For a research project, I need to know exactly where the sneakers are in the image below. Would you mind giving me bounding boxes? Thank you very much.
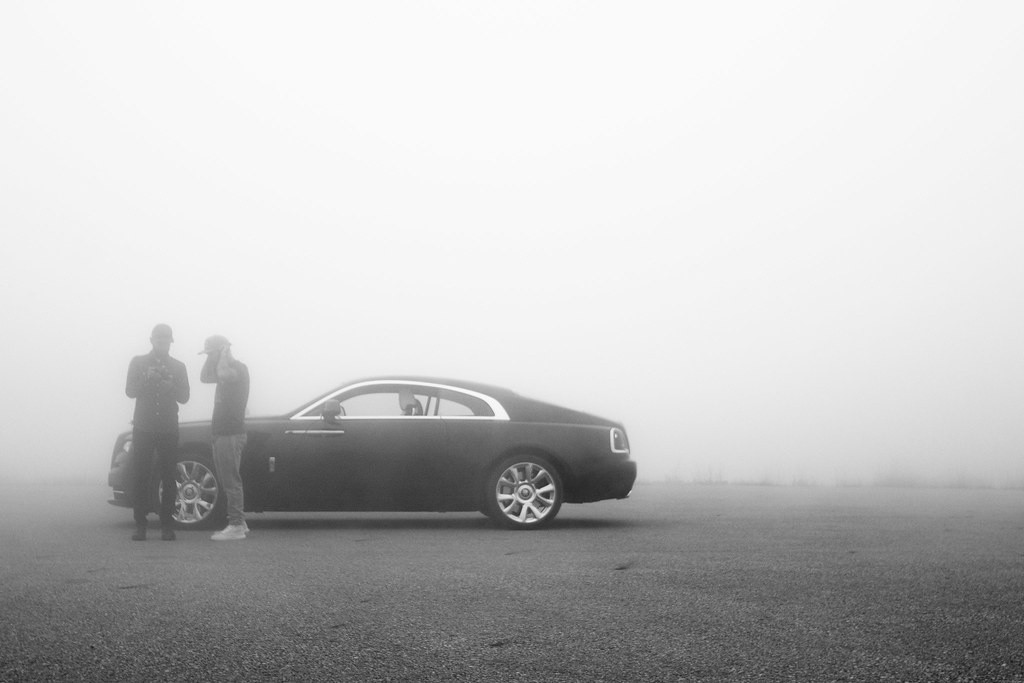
[210,521,249,540]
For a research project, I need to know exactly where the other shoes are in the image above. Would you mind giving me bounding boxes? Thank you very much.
[131,532,145,540]
[162,530,176,540]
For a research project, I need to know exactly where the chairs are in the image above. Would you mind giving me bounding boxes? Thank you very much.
[398,388,417,416]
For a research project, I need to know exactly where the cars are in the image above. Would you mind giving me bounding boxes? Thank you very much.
[111,375,639,533]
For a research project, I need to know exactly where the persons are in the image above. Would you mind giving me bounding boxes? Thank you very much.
[125,324,191,539]
[197,336,249,538]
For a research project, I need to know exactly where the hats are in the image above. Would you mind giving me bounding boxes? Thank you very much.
[151,324,173,342]
[198,334,231,355]
